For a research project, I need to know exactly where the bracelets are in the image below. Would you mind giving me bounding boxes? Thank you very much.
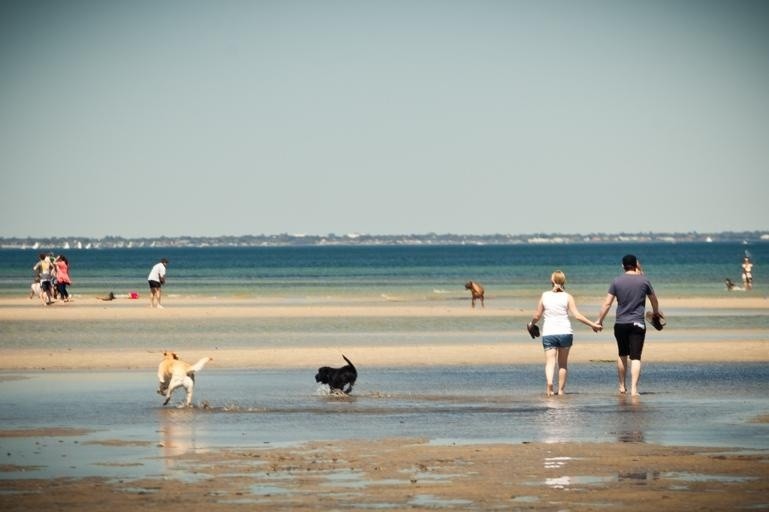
[531,320,536,326]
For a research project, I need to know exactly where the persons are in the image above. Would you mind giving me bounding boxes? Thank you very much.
[741,258,755,291]
[147,258,169,309]
[725,278,747,291]
[95,291,140,301]
[527,269,604,396]
[590,254,665,397]
[28,250,74,307]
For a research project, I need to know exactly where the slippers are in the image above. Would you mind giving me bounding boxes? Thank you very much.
[646,309,666,330]
[527,321,540,338]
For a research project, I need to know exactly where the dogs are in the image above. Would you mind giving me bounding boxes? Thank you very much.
[463,279,487,309]
[314,353,359,398]
[153,348,211,407]
[30,280,57,304]
